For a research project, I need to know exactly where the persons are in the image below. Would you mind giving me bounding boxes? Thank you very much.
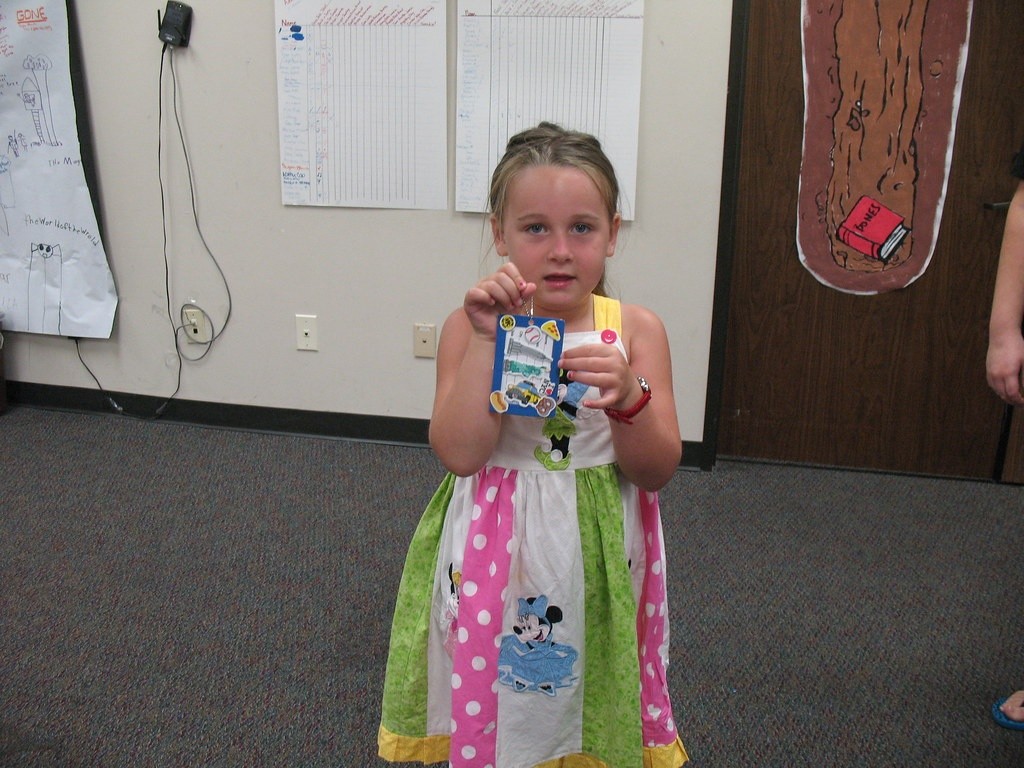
[983,149,1024,732]
[373,121,694,767]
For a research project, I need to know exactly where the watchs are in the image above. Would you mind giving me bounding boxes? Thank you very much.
[602,375,653,426]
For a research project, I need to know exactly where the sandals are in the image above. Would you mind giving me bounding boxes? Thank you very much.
[992,690,1024,730]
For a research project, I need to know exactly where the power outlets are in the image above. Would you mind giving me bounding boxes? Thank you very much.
[182,310,207,344]
[296,315,318,350]
[414,323,436,358]
